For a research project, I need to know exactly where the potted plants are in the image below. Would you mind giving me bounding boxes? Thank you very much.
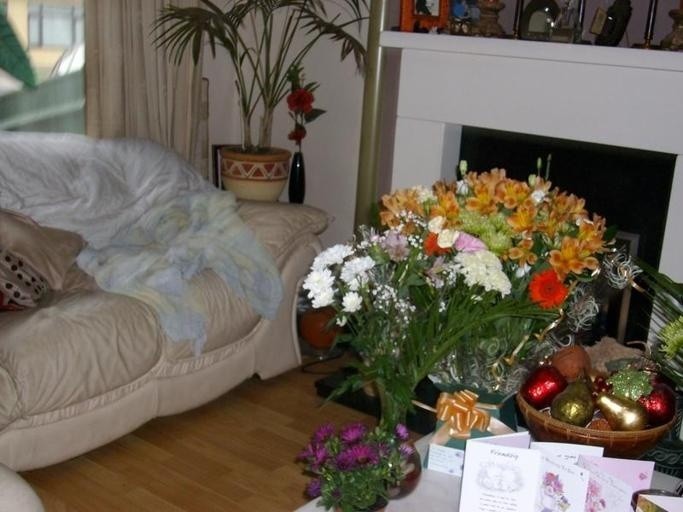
[152,1,374,204]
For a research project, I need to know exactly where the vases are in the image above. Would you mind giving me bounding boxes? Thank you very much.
[289,150,306,204]
[374,424,424,501]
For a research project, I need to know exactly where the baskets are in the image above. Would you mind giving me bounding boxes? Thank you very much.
[517,383,679,458]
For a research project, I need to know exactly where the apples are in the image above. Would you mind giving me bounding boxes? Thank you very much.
[640,386,675,424]
[521,366,567,410]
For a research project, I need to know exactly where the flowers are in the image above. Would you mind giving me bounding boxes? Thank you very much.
[306,211,542,430]
[279,64,317,149]
[295,419,415,510]
[629,255,682,388]
[381,156,614,467]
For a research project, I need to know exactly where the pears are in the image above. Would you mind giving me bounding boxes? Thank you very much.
[593,389,649,430]
[551,379,594,426]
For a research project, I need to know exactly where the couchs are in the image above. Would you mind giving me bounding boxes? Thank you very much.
[1,126,326,476]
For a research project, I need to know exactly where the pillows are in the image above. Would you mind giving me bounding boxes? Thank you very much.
[1,203,94,304]
